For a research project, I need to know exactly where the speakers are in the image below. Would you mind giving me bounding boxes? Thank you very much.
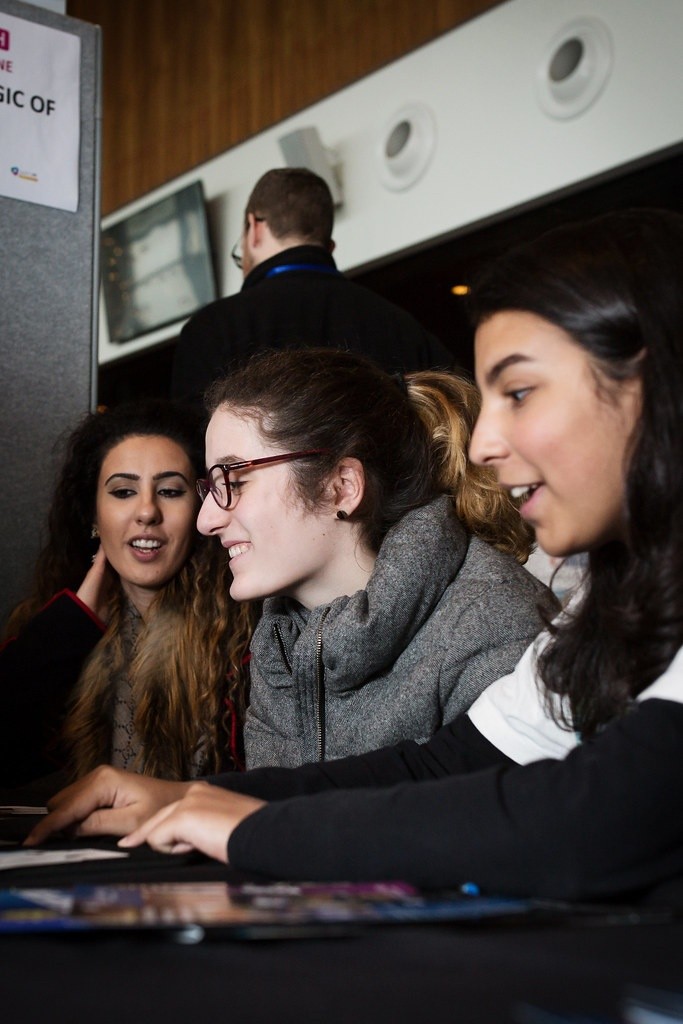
[279,128,344,206]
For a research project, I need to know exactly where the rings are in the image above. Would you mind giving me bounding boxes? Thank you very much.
[90,554,98,564]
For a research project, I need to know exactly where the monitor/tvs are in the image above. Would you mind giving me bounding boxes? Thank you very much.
[100,180,221,344]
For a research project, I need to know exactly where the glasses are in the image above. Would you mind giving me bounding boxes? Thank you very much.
[195,448,333,510]
[231,216,266,269]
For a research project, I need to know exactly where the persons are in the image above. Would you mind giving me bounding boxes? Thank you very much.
[1,206,682,916]
[156,168,453,439]
